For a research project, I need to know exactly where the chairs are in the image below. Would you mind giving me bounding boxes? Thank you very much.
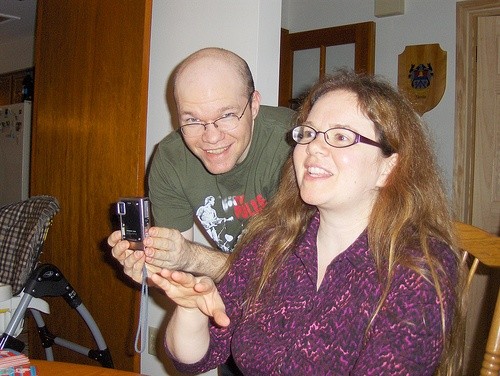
[449,222,500,376]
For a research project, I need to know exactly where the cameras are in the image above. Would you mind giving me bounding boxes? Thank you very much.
[118,197,151,242]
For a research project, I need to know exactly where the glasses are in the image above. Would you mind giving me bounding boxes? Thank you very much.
[178,93,253,137]
[291,123,386,150]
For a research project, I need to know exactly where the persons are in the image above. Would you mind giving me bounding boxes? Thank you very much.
[152,69,468,376]
[107,47,298,376]
